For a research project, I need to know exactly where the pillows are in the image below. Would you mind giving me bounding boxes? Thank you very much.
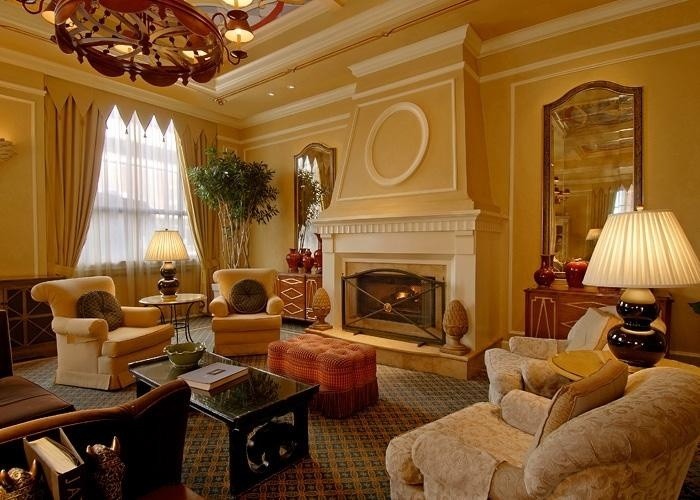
[227,279,268,313]
[534,359,627,446]
[563,307,623,351]
[76,291,124,332]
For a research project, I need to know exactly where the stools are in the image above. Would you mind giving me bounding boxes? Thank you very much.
[267,333,379,418]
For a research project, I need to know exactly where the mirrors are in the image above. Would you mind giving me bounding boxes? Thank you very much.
[542,79,643,278]
[294,143,338,258]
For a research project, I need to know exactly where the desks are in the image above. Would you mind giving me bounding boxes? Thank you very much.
[137,486,204,500]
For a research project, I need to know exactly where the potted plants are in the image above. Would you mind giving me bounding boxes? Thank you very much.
[294,163,327,249]
[188,146,279,299]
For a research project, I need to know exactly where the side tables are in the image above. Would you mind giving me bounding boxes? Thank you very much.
[139,294,207,344]
[550,351,697,382]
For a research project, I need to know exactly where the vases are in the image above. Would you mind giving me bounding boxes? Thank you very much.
[285,247,322,275]
[564,262,587,287]
[533,255,555,289]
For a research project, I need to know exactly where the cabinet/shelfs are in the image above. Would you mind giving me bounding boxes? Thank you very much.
[524,287,675,359]
[277,273,322,323]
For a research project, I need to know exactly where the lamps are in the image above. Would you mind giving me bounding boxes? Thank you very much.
[554,176,570,204]
[581,208,700,367]
[587,229,602,246]
[144,228,190,296]
[15,1,252,86]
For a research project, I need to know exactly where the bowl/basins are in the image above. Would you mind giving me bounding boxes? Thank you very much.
[163,342,206,366]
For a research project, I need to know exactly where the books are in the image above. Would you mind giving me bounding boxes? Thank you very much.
[21,427,87,500]
[176,361,249,390]
[189,375,251,397]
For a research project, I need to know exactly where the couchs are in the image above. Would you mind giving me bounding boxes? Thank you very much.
[31,276,175,390]
[210,269,284,356]
[383,361,700,499]
[483,304,668,402]
[1,310,190,487]
